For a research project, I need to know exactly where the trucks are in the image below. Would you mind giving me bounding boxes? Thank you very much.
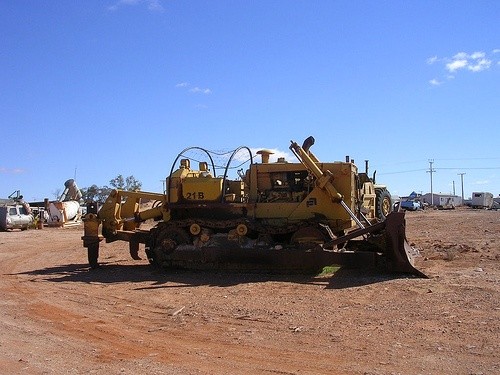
[401,196,422,211]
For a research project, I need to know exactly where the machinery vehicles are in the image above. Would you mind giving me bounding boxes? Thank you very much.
[81,136,429,279]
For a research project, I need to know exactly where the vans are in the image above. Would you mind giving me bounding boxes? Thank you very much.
[0,204,35,232]
